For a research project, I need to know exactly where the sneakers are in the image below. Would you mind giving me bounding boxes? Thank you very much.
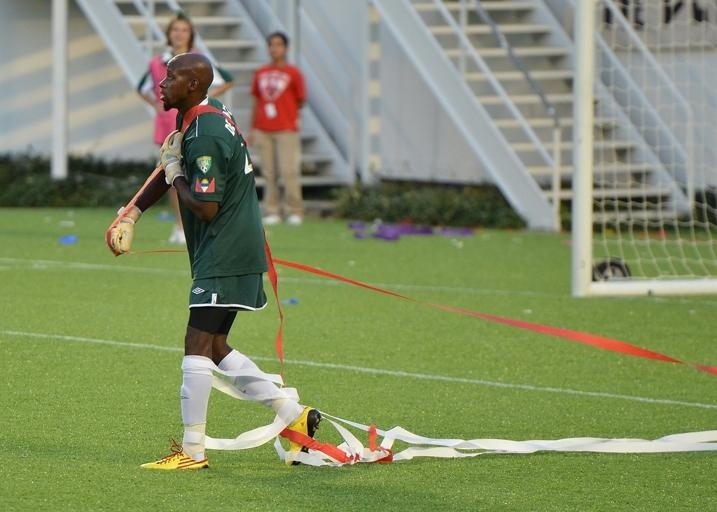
[286,405,321,466]
[262,213,303,225]
[170,226,185,243]
[141,450,209,470]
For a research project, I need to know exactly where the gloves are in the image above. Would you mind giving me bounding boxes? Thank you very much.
[106,204,143,257]
[159,129,184,187]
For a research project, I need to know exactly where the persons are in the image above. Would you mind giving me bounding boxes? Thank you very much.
[134,15,235,242]
[105,53,322,470]
[246,32,308,224]
